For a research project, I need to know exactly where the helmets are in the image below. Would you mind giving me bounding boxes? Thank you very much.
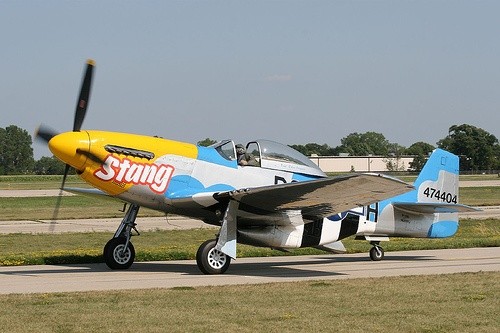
[233,144,246,155]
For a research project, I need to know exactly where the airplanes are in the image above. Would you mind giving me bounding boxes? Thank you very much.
[35,59,482,275]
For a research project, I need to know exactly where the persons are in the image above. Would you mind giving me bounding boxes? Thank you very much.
[235,144,260,167]
